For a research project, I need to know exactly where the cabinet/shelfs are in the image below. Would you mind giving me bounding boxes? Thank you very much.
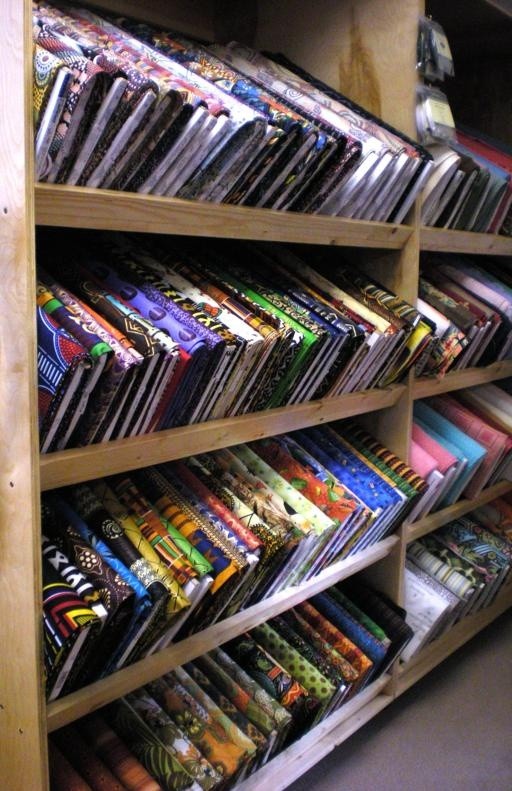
[0,0,511,791]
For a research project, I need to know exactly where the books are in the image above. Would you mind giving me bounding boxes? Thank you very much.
[44,378,511,791]
[36,226,510,458]
[30,1,512,240]
